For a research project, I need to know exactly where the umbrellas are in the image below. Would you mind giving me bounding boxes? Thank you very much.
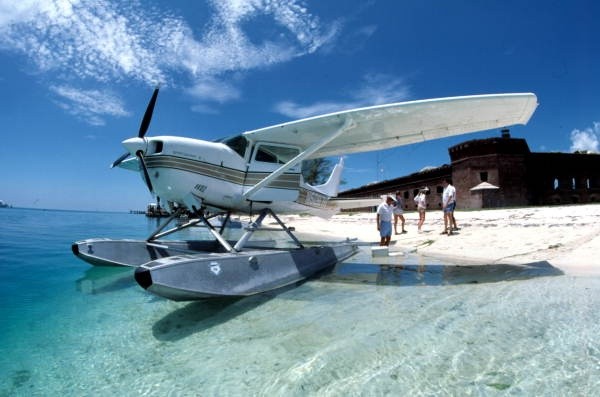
[469,182,500,208]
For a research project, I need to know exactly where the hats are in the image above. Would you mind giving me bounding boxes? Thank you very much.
[388,195,398,202]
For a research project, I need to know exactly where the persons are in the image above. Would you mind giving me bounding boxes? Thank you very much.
[393,190,408,235]
[377,194,396,247]
[447,179,459,231]
[413,185,430,233]
[440,178,454,236]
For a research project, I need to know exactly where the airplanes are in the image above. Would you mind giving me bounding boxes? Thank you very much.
[72,81,538,303]
